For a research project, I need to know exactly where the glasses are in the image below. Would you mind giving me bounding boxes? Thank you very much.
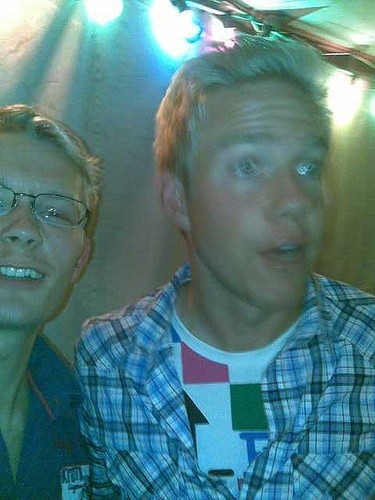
[1,185,91,230]
